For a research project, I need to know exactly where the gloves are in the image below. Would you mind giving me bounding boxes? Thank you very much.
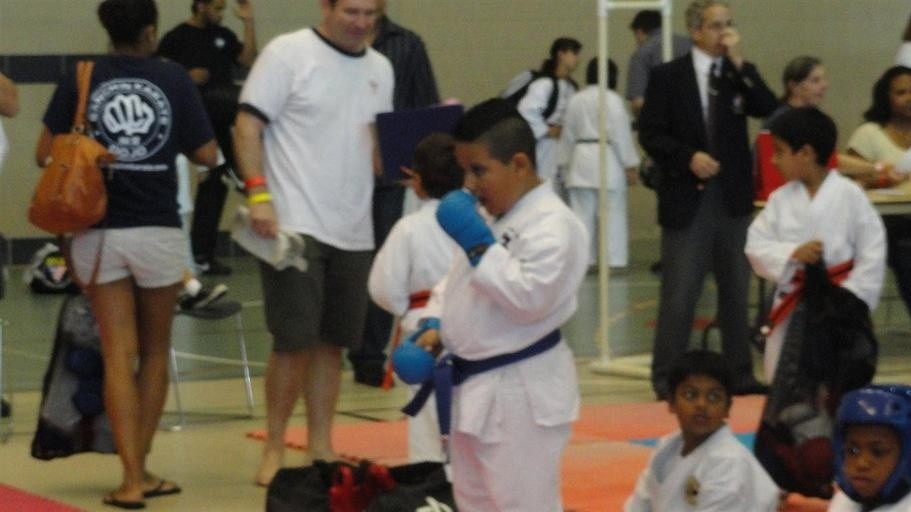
[432,187,498,272]
[390,314,444,389]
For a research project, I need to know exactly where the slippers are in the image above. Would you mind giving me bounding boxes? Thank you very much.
[103,489,146,509]
[144,470,183,497]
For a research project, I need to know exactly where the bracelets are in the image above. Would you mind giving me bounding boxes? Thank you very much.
[244,191,275,208]
[874,159,884,173]
[242,176,271,188]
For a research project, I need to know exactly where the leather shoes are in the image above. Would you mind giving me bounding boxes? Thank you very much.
[732,382,772,396]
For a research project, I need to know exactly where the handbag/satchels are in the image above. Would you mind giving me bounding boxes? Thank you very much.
[22,59,118,235]
[25,284,128,461]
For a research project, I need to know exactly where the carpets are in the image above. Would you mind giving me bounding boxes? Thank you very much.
[0,483,86,512]
[245,394,840,512]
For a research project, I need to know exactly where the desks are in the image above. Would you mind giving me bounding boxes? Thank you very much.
[754,190,911,318]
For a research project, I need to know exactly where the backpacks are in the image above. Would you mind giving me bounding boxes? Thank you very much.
[498,70,561,120]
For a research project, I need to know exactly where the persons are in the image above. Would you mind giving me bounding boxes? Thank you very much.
[2,0,911,512]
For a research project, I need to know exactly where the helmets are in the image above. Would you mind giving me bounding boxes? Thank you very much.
[831,382,910,511]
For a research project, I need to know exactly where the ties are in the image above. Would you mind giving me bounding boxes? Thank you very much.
[705,59,724,148]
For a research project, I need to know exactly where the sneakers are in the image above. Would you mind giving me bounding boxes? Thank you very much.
[342,345,397,390]
[180,281,233,314]
[253,441,290,490]
[302,441,348,465]
[191,252,238,282]
